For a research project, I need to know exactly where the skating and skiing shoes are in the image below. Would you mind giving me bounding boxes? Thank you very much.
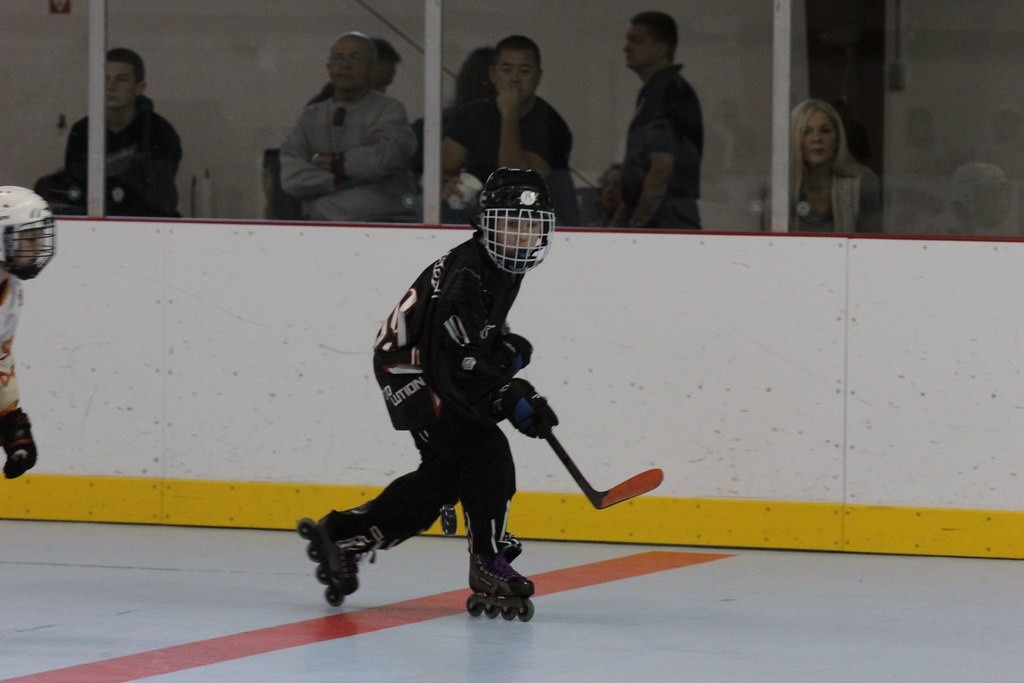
[466,552,535,622]
[298,509,359,606]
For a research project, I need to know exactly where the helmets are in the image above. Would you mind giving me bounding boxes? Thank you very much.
[478,168,555,274]
[0,185,56,280]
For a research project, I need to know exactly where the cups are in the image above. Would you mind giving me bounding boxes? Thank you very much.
[448,172,483,211]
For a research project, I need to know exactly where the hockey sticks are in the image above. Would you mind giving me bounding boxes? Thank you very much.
[548,432,664,511]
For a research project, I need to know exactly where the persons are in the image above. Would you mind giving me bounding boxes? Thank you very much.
[263,41,401,218]
[440,35,580,224]
[606,11,704,230]
[63,47,181,217]
[789,99,882,233]
[414,47,494,173]
[295,166,560,621]
[601,164,623,213]
[279,30,419,222]
[1,185,55,478]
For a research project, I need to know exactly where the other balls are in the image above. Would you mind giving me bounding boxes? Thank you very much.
[440,503,458,537]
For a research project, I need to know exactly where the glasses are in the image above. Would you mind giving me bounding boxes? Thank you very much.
[329,55,371,65]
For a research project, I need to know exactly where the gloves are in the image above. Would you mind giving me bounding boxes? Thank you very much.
[481,334,533,377]
[0,408,38,479]
[496,378,558,439]
